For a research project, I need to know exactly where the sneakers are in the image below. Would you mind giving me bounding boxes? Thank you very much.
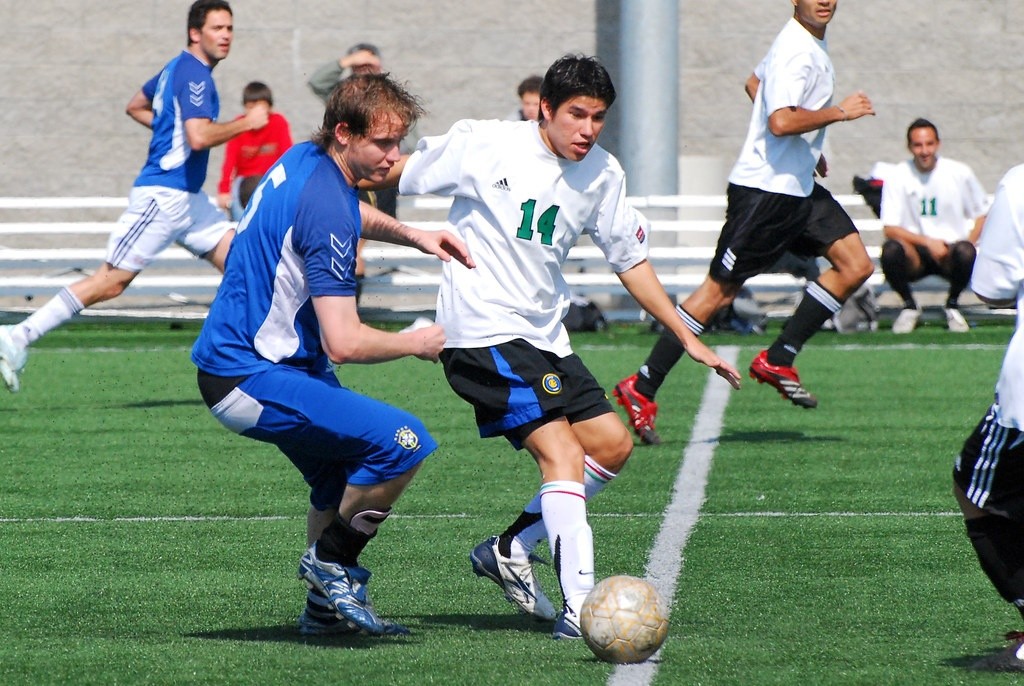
[551,610,584,640]
[893,304,922,336]
[613,372,662,447]
[943,305,968,334]
[297,612,399,637]
[0,325,27,392]
[296,539,385,635]
[748,351,817,408]
[973,630,1024,669]
[469,533,558,621]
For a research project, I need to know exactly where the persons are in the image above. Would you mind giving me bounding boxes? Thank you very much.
[0,0,268,395]
[952,163,1024,660]
[355,53,741,637]
[506,74,544,123]
[613,0,876,447]
[309,42,421,310]
[218,82,291,222]
[880,119,986,333]
[193,69,474,636]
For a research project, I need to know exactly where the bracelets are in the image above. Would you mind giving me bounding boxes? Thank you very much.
[834,104,848,121]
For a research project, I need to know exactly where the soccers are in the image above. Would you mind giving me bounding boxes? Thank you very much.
[579,575,669,664]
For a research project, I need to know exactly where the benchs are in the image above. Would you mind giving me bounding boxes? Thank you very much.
[0,195,994,291]
